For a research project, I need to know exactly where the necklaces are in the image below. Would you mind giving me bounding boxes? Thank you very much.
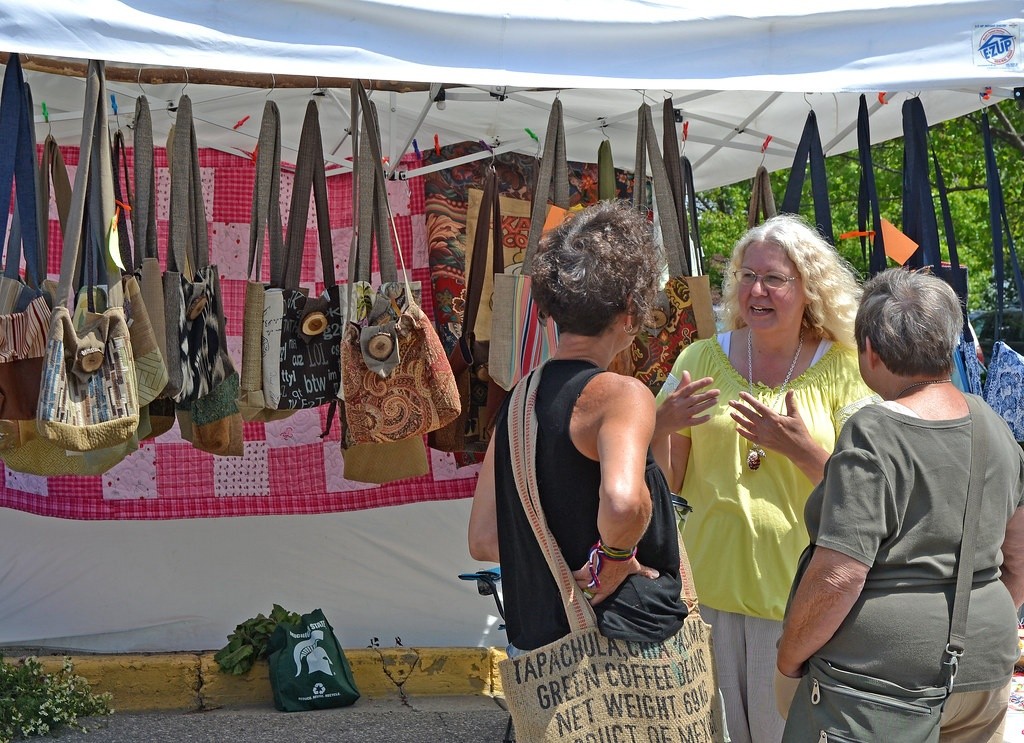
[891,379,953,401]
[748,327,804,469]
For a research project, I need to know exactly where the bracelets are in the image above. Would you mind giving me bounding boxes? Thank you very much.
[588,539,637,587]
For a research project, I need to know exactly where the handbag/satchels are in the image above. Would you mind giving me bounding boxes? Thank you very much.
[0,53,717,484]
[498,614,726,743]
[781,656,950,743]
[267,608,360,712]
[902,97,1024,442]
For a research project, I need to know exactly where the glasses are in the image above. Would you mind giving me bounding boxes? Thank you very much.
[732,267,801,289]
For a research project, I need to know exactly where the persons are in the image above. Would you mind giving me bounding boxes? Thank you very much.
[650,215,883,743]
[776,270,1024,743]
[468,201,689,655]
[941,263,985,361]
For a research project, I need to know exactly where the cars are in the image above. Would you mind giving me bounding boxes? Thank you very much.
[963,306,1024,357]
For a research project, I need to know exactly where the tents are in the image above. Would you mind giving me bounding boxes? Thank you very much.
[0,0,1024,654]
